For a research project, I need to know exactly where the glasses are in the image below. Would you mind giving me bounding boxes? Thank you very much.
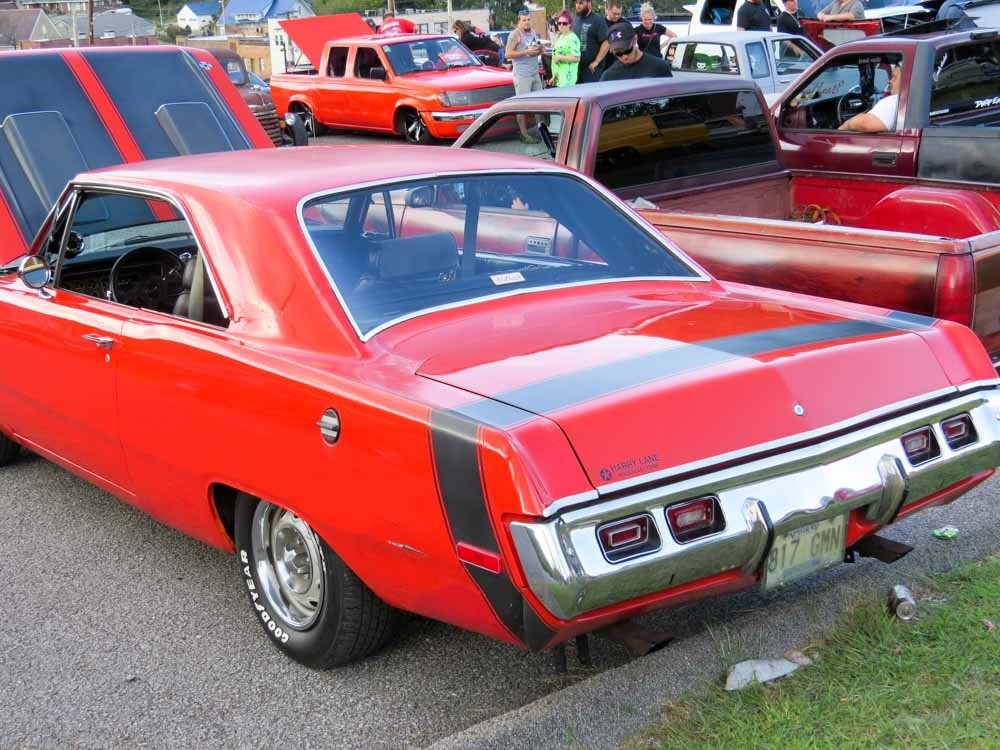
[454,31,456,33]
[614,42,635,57]
[558,21,568,26]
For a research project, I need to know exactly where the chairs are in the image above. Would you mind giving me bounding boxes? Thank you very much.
[409,56,437,71]
[170,252,224,320]
[365,230,460,277]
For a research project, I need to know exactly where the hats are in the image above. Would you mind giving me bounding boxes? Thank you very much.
[607,22,634,54]
[517,7,530,17]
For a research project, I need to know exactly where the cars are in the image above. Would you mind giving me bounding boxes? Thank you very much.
[267,12,539,144]
[438,0,1000,149]
[1,43,1000,672]
[202,45,311,147]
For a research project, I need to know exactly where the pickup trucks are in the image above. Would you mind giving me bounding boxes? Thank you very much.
[311,72,999,381]
[767,27,1000,188]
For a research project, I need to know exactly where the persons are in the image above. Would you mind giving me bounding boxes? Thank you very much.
[547,8,581,88]
[504,7,550,145]
[452,21,505,66]
[572,0,609,84]
[836,60,903,133]
[603,0,631,69]
[599,22,673,82]
[634,2,677,60]
[738,0,776,73]
[777,0,803,58]
[377,11,417,33]
[817,0,864,22]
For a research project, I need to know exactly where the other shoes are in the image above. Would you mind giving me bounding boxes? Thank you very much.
[520,135,538,144]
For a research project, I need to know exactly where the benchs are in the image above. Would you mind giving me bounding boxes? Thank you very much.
[354,262,607,288]
